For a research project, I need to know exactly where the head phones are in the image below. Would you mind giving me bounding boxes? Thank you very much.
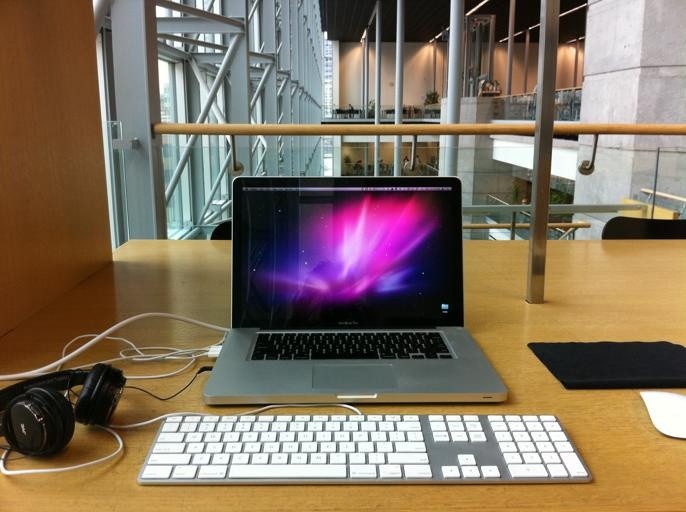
[0,364,127,457]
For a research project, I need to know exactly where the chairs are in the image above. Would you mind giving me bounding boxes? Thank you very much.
[329,102,422,119]
[602,214,685,239]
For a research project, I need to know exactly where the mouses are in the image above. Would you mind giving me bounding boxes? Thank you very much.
[639,389,685,437]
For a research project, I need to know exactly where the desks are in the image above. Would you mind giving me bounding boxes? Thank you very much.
[0,239,686,512]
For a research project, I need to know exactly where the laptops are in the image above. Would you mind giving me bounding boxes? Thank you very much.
[203,174,508,406]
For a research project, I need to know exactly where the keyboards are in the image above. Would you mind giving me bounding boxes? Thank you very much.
[138,412,592,484]
